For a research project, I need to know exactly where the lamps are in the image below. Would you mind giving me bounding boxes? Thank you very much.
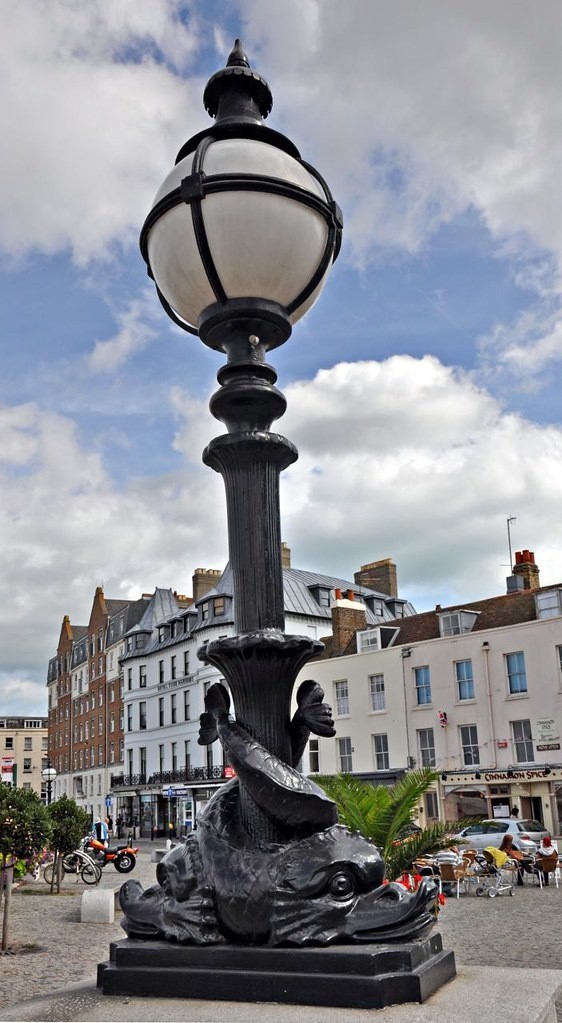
[441,768,449,780]
[544,762,553,774]
[475,768,484,779]
[507,764,514,777]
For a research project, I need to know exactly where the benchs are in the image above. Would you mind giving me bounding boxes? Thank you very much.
[151,849,169,861]
[79,881,124,925]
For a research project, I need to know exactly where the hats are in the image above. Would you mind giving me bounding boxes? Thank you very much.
[543,837,551,847]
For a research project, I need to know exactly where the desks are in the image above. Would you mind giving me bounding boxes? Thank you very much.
[519,857,534,864]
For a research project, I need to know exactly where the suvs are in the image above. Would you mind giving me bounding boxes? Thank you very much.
[453,818,551,854]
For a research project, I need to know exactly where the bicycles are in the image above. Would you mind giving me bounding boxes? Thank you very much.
[44,846,102,886]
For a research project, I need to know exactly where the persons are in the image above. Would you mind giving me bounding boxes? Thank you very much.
[93,817,108,845]
[499,835,558,886]
[413,846,462,896]
[105,815,113,847]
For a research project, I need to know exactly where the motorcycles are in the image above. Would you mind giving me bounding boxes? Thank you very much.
[62,835,140,873]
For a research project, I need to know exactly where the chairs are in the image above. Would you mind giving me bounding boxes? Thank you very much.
[414,846,562,899]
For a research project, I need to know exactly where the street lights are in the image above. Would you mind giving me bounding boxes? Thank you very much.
[96,38,456,1009]
[40,758,57,806]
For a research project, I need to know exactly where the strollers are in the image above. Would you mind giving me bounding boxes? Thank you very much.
[474,847,516,898]
